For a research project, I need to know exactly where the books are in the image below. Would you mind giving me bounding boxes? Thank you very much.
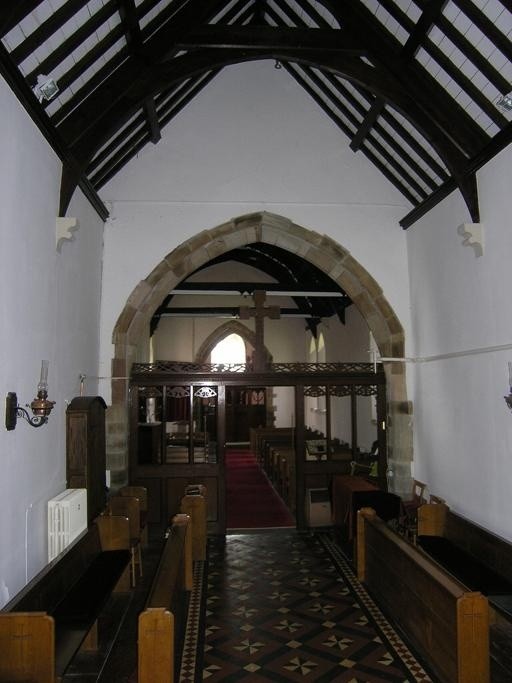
[47,487,89,562]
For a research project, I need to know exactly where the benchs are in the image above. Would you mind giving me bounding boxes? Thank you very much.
[416,503,511,600]
[249,425,359,513]
[0,518,133,683]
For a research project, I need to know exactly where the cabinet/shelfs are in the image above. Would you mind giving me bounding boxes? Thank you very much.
[66,396,107,523]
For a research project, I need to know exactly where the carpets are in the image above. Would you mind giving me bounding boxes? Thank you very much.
[225,448,296,528]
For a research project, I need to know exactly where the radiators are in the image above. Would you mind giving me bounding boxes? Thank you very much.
[47,488,88,565]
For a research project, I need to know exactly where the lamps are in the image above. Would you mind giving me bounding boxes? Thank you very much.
[6,359,56,431]
[497,91,512,110]
[33,74,59,105]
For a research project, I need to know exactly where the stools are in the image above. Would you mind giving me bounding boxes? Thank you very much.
[129,538,143,587]
